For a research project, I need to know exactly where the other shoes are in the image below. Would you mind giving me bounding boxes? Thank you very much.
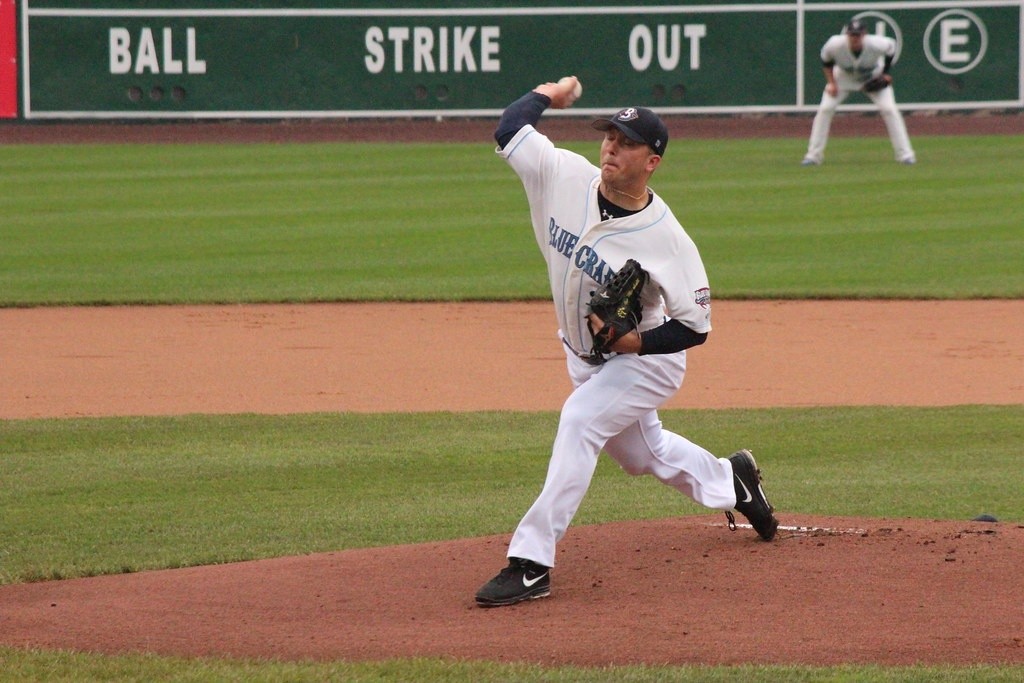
[801,159,818,166]
[904,157,916,165]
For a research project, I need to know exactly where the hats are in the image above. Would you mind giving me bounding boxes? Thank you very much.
[592,108,668,156]
[847,21,864,35]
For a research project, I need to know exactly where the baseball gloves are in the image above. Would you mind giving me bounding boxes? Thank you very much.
[583,259,650,356]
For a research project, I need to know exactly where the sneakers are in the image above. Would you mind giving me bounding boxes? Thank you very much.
[724,449,776,541]
[475,557,551,605]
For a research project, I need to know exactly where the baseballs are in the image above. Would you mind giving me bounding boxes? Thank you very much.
[557,76,582,102]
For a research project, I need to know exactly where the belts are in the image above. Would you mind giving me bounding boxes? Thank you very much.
[562,339,624,366]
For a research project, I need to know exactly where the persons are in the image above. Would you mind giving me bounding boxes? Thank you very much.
[801,20,915,165]
[474,76,778,607]
